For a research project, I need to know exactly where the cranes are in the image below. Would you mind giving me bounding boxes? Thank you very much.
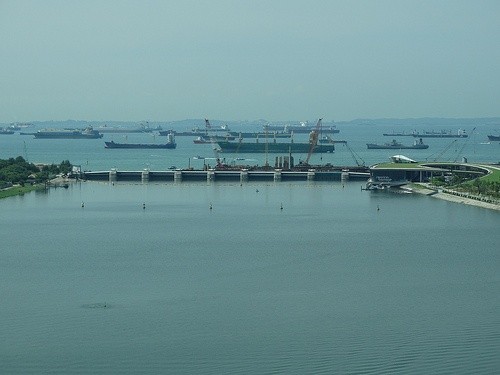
[304,118,323,163]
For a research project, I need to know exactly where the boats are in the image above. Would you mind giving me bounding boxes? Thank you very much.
[487,135,500,141]
[216,131,335,154]
[319,135,348,144]
[104,132,178,149]
[382,127,468,138]
[0,116,340,144]
[365,137,429,149]
